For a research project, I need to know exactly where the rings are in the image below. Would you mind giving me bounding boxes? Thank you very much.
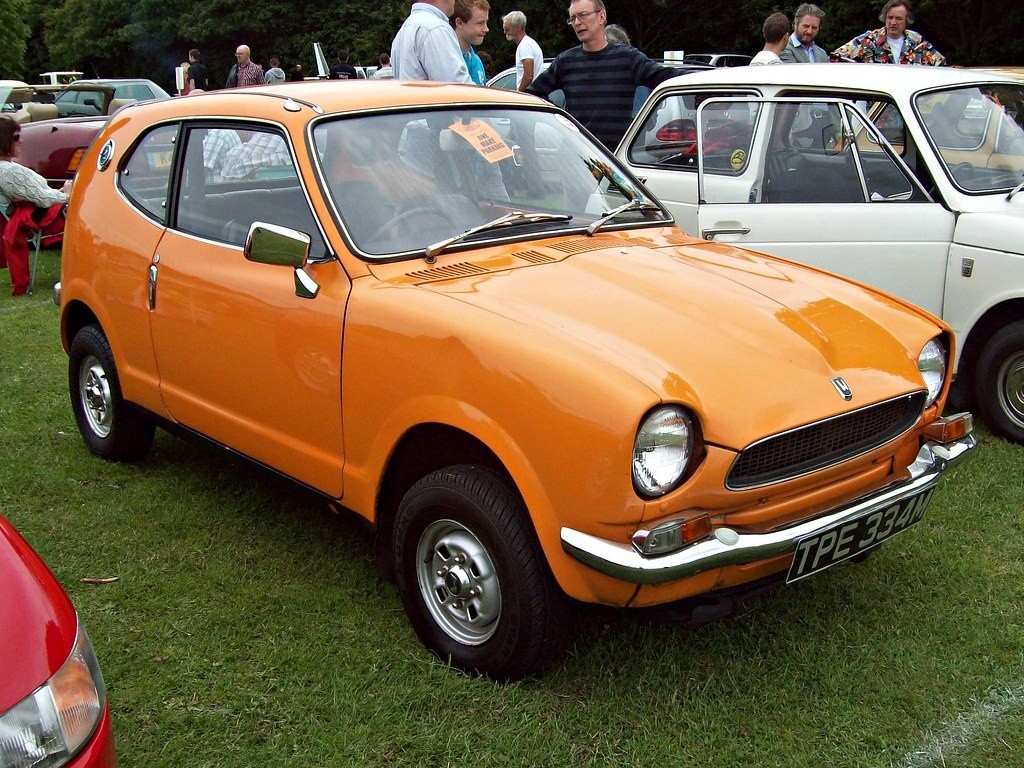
[70,182,73,183]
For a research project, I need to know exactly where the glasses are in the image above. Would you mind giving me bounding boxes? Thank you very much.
[13,134,20,142]
[235,52,248,57]
[567,10,601,25]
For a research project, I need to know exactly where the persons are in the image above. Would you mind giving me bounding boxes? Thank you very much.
[329,50,357,79]
[780,4,830,149]
[226,45,265,88]
[61,75,76,84]
[187,49,209,92]
[181,62,191,96]
[373,54,392,79]
[605,24,650,147]
[501,11,544,199]
[0,116,73,208]
[449,0,490,87]
[391,0,511,202]
[746,13,801,143]
[265,58,285,84]
[203,129,292,182]
[832,0,946,128]
[525,0,695,211]
[477,51,491,70]
[291,65,304,81]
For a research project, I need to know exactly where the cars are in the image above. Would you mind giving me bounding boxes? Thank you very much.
[51,77,978,694]
[584,63,1024,448]
[475,53,1023,187]
[0,71,186,190]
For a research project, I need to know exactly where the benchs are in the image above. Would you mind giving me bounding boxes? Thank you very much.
[694,150,820,203]
[147,184,384,257]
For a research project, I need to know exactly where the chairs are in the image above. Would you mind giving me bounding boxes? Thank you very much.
[107,99,137,115]
[27,102,59,121]
[0,185,68,296]
[375,194,481,236]
[833,159,914,200]
